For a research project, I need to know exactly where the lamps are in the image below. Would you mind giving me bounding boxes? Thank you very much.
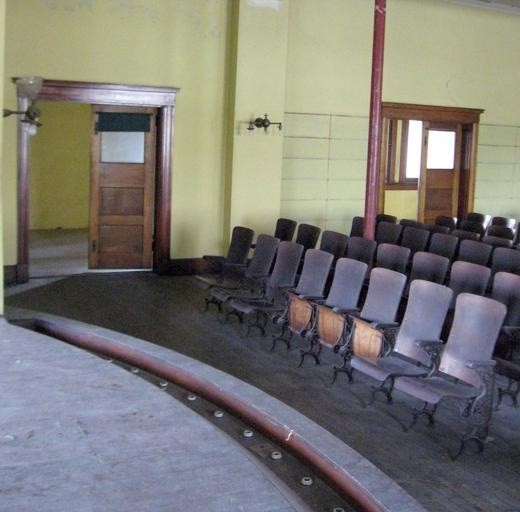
[3,76,44,127]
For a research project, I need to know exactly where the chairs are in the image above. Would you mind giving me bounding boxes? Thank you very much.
[393,292,507,459]
[195,212,520,408]
